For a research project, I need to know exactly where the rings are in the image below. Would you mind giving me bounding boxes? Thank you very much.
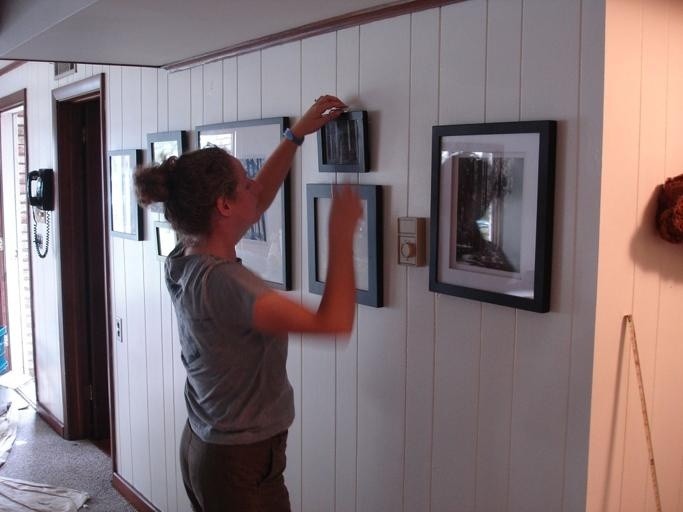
[313,97,319,103]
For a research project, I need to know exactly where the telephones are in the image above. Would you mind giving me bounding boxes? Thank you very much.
[26,168,55,213]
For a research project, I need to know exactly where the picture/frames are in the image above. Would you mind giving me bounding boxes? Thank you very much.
[428,118,558,313]
[146,130,185,212]
[195,116,292,292]
[153,222,180,261]
[306,182,383,308]
[317,109,371,170]
[106,148,144,242]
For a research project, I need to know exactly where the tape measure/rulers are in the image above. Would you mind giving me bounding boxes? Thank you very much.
[625,312,673,506]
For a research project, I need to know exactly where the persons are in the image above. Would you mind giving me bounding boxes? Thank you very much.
[132,95,363,511]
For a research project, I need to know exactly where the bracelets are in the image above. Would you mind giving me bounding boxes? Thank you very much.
[282,127,305,147]
